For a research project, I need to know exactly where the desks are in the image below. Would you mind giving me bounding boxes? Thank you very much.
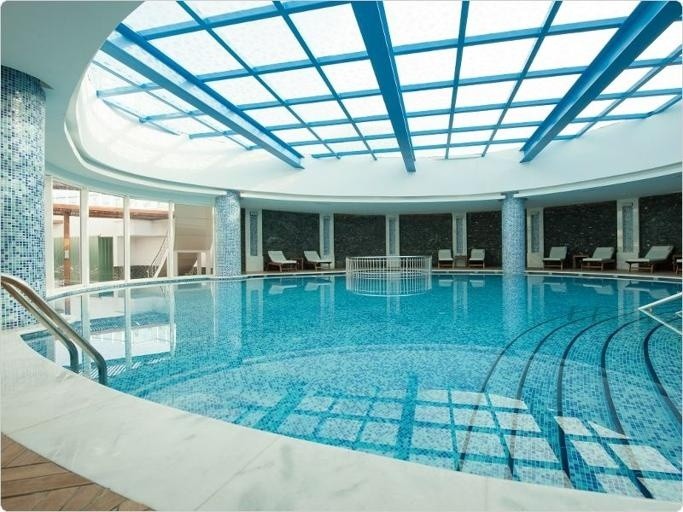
[289,257,304,271]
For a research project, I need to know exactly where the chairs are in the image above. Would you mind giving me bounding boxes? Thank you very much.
[269,283,297,296]
[302,281,332,292]
[626,281,670,300]
[438,279,453,288]
[266,251,297,273]
[542,245,682,276]
[583,280,614,296]
[543,283,567,293]
[468,249,486,269]
[438,249,454,269]
[304,251,332,272]
[468,280,486,288]
